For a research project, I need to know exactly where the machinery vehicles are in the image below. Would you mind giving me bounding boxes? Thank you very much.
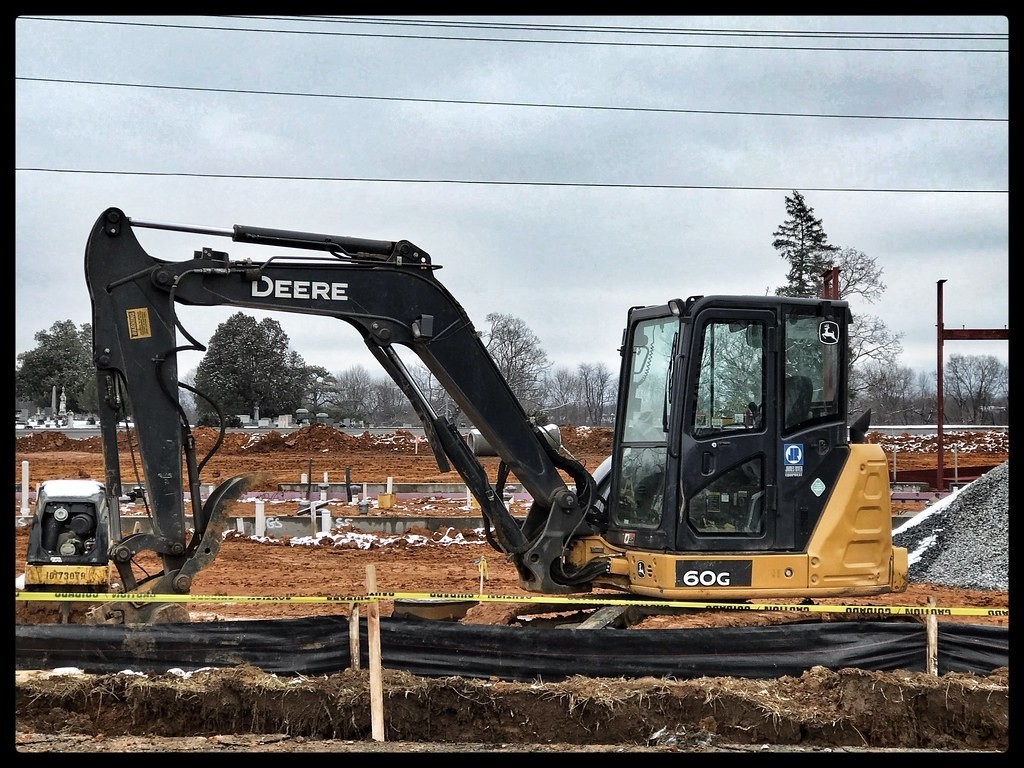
[82,206,911,628]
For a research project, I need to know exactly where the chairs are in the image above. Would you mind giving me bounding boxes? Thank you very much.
[715,376,813,492]
